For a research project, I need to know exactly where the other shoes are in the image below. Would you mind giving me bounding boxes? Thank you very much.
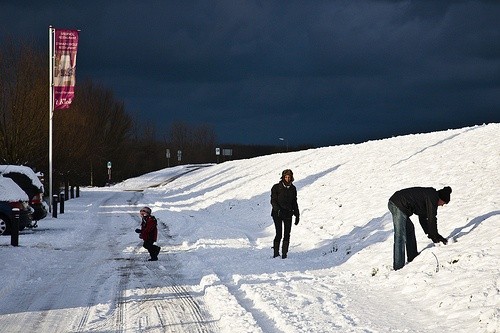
[148,256,158,261]
[153,245,160,256]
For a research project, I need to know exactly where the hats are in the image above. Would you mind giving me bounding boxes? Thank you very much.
[281,170,294,185]
[140,207,151,215]
[436,187,452,204]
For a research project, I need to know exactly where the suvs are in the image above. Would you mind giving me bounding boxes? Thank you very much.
[0,165,48,236]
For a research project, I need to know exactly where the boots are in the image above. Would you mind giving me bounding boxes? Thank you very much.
[282,241,289,259]
[274,239,281,258]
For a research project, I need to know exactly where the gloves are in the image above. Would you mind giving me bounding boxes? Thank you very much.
[294,210,299,225]
[428,233,439,243]
[135,229,141,233]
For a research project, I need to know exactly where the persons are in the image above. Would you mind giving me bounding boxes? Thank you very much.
[388,186,452,271]
[270,170,299,259]
[135,206,161,261]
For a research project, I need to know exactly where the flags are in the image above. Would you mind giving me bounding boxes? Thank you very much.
[54,29,78,109]
